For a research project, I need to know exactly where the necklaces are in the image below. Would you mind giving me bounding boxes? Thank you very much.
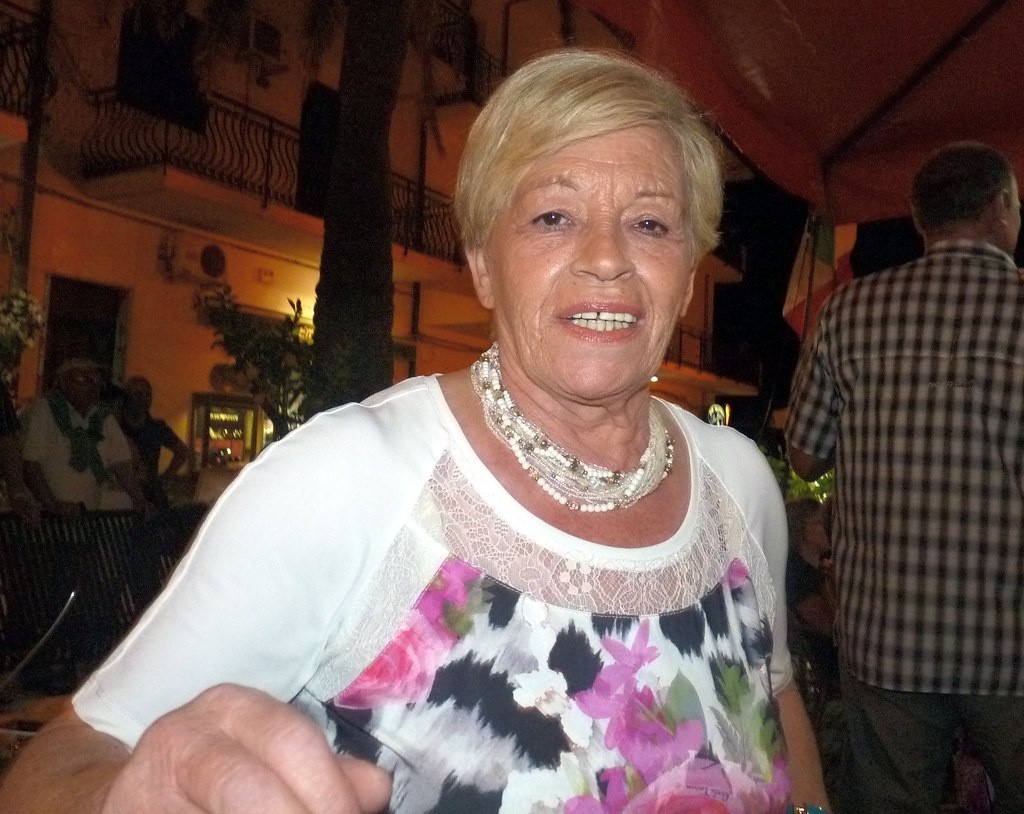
[470,343,676,512]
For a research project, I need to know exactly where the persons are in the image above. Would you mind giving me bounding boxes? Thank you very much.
[784,145,1024,814]
[0,354,188,526]
[0,50,836,814]
[786,496,837,691]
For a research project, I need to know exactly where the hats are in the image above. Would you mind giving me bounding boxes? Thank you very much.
[55,358,101,375]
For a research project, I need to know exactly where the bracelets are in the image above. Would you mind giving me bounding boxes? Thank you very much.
[786,803,826,814]
